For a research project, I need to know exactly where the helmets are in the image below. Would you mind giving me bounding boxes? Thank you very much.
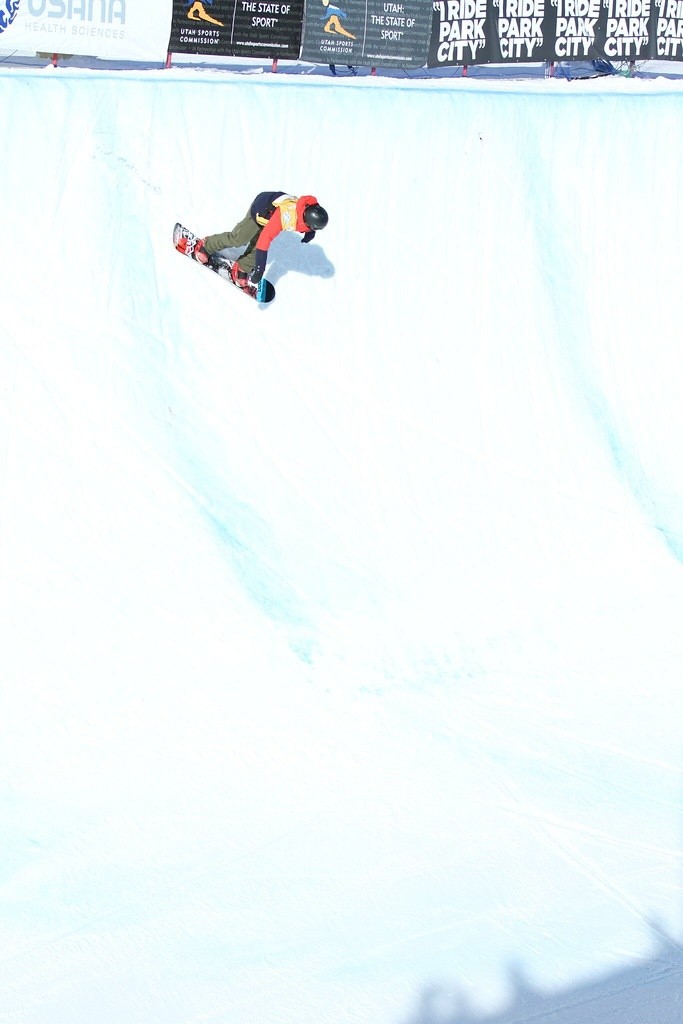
[305,205,328,230]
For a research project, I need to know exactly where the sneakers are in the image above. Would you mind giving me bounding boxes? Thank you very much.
[196,237,208,264]
[234,261,248,287]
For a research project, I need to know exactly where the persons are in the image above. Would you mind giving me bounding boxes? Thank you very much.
[196,191,329,287]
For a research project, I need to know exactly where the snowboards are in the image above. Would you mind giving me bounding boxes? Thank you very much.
[172,223,275,303]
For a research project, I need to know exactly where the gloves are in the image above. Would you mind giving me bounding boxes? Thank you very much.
[301,231,315,243]
[251,270,263,283]
